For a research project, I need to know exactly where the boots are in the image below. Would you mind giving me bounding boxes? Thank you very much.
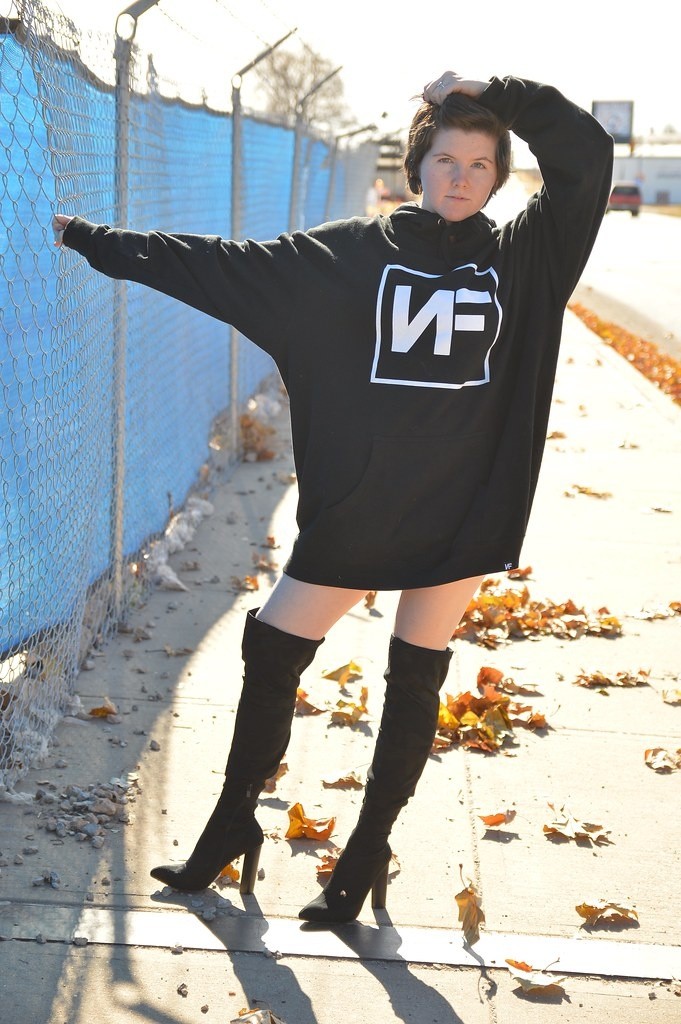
[152,610,324,900]
[299,637,453,929]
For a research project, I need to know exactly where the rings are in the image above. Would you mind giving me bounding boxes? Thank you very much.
[434,80,447,90]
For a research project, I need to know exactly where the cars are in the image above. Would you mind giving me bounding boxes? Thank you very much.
[603,182,641,216]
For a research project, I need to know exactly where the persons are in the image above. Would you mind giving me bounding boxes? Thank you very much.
[48,68,617,930]
